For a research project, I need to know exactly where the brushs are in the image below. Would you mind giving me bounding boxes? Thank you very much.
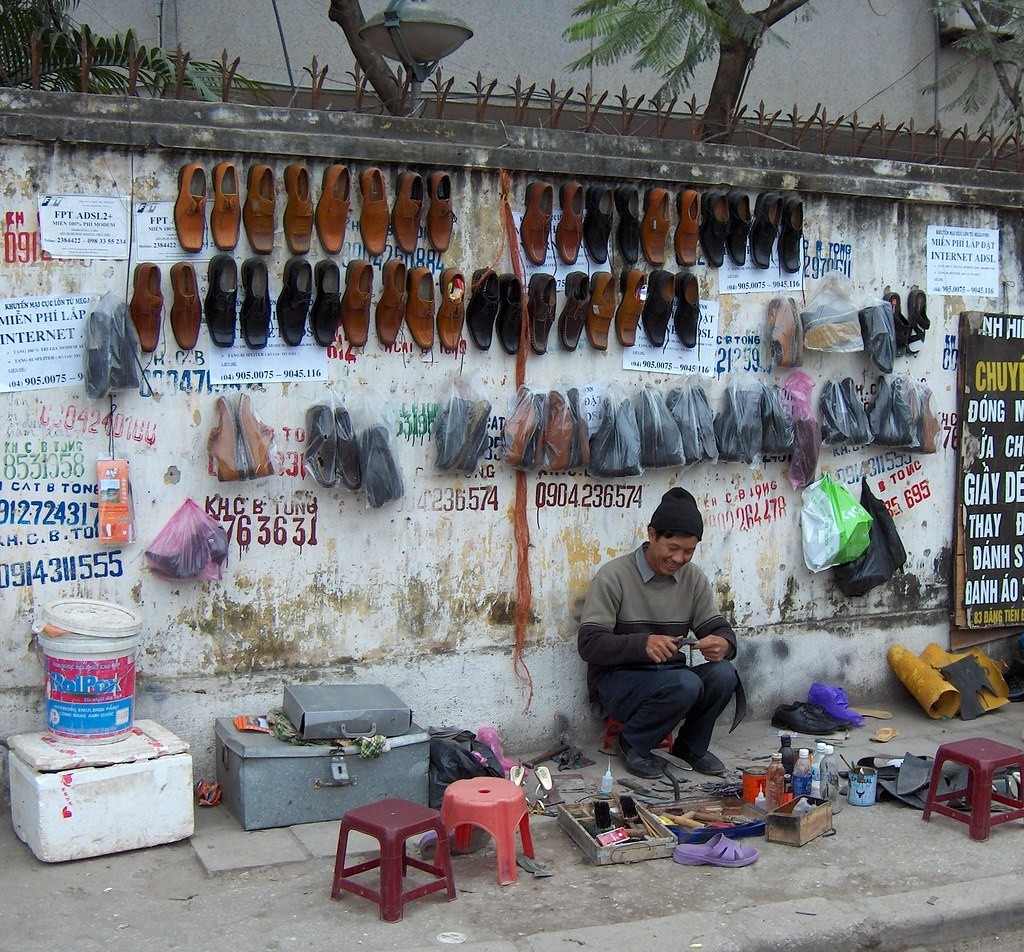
[619,796,641,822]
[593,801,614,831]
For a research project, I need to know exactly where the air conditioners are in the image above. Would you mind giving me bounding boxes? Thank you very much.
[938,0,1019,39]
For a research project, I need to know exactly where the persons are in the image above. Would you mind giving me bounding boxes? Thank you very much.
[577,487,738,778]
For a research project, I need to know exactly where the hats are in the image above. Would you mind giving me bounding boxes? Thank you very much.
[647,487,704,542]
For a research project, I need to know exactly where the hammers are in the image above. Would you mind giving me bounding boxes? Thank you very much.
[519,743,571,769]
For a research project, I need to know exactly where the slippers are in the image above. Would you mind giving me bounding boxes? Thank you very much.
[883,290,929,357]
[869,728,899,743]
[673,832,758,868]
[419,823,492,860]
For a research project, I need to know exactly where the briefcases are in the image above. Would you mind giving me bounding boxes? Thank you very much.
[282,685,412,740]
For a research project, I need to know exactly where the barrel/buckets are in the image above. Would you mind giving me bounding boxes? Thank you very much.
[847,766,878,806]
[742,764,769,801]
[35,605,141,746]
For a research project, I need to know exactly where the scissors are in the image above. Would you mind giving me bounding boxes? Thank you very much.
[675,638,697,650]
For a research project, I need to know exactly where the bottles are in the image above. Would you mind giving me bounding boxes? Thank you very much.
[765,751,784,811]
[811,744,826,798]
[601,770,613,792]
[778,734,794,777]
[792,748,812,797]
[754,792,766,810]
[820,744,840,814]
[783,774,794,804]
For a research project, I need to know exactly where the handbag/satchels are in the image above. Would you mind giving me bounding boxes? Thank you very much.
[799,473,873,574]
[832,476,907,596]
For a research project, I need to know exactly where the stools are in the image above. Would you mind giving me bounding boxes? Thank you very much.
[921,737,1024,844]
[601,715,673,756]
[326,796,458,923]
[431,774,537,887]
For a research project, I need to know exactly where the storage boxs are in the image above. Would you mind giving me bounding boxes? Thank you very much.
[282,682,415,737]
[212,715,433,833]
[556,798,678,868]
[5,717,197,863]
[764,793,835,848]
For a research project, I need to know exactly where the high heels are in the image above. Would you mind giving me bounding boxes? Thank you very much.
[510,765,525,790]
[1005,771,1022,799]
[534,765,566,815]
[145,526,230,580]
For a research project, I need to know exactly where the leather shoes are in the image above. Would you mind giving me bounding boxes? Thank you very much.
[305,405,363,491]
[86,303,139,401]
[206,394,274,483]
[1002,659,1024,702]
[128,164,895,374]
[616,733,665,779]
[672,741,725,775]
[434,376,939,489]
[357,424,406,509]
[771,702,853,736]
